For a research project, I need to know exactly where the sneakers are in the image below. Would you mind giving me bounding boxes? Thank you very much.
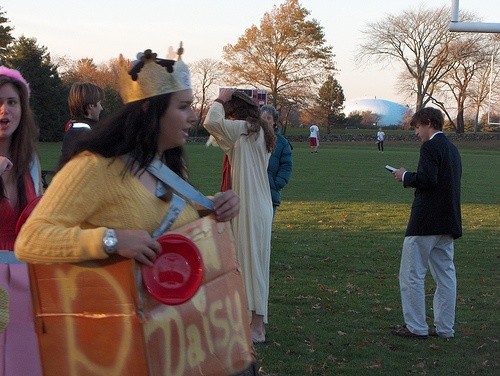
[391,325,427,338]
[428,328,438,337]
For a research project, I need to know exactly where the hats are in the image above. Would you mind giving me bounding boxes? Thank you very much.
[0,66,30,98]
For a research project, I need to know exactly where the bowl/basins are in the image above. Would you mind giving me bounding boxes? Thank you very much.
[142,233,205,306]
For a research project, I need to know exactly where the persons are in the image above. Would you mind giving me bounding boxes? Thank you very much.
[310,124,320,153]
[392,108,463,337]
[59,82,104,168]
[376,128,386,153]
[204,88,274,343]
[259,105,292,217]
[0,65,42,376]
[15,41,240,376]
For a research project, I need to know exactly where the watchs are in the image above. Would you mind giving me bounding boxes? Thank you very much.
[103,229,118,255]
